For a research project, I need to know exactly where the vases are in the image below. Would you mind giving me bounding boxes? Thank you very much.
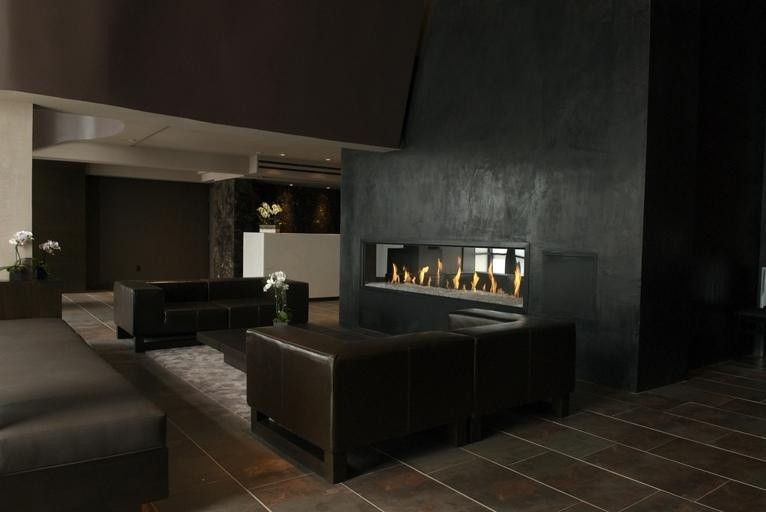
[274,319,289,327]
[34,268,48,280]
[260,225,280,234]
[10,270,29,282]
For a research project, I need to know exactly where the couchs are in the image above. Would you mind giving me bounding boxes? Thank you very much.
[246,309,576,484]
[113,277,308,352]
[0,281,169,511]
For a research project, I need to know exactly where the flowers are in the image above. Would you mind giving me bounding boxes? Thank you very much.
[0,231,37,272]
[38,240,62,269]
[263,271,294,322]
[258,203,283,225]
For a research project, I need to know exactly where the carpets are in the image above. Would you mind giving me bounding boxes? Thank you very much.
[144,345,274,422]
